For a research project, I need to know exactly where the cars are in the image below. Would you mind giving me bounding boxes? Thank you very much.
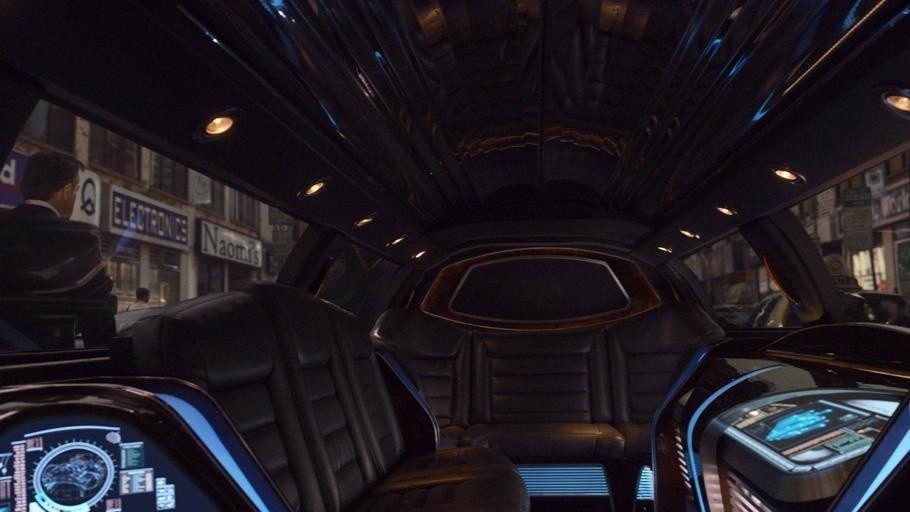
[751,291,906,327]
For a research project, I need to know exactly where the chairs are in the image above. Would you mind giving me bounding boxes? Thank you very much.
[109,283,728,512]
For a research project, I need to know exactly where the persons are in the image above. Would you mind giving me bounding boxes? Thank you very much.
[0,151,119,349]
[126,286,151,312]
